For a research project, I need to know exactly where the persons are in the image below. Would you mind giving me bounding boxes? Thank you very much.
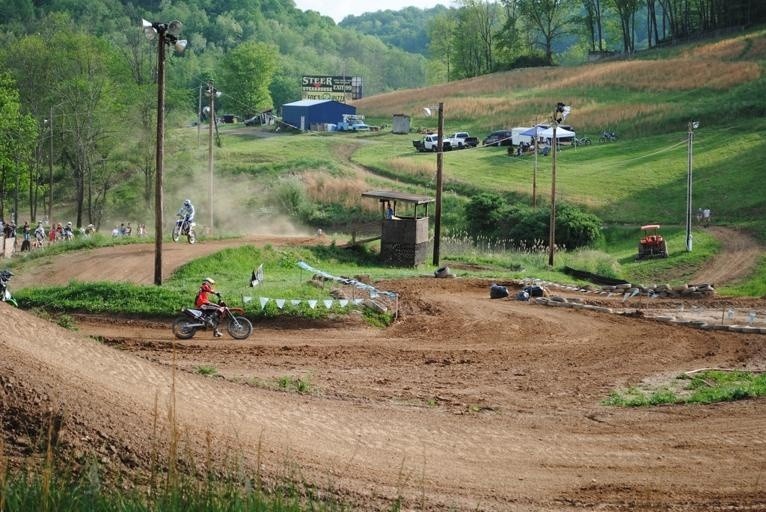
[177,199,195,237]
[696,208,704,224]
[703,207,710,228]
[384,204,394,220]
[0,220,148,248]
[195,278,224,337]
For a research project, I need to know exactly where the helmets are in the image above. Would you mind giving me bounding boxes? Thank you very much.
[201,277,214,288]
[183,199,189,207]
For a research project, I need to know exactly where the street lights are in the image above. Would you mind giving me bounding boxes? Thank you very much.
[202,79,222,228]
[534,103,571,266]
[142,20,188,283]
[687,118,700,252]
[424,102,444,267]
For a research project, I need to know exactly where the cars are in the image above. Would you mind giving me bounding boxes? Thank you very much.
[244,116,270,126]
[343,119,369,131]
[412,131,512,151]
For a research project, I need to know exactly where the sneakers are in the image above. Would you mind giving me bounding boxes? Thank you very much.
[205,316,213,326]
[213,332,222,337]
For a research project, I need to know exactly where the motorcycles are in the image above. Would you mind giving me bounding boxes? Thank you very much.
[575,132,619,145]
[172,292,253,340]
[172,208,196,244]
